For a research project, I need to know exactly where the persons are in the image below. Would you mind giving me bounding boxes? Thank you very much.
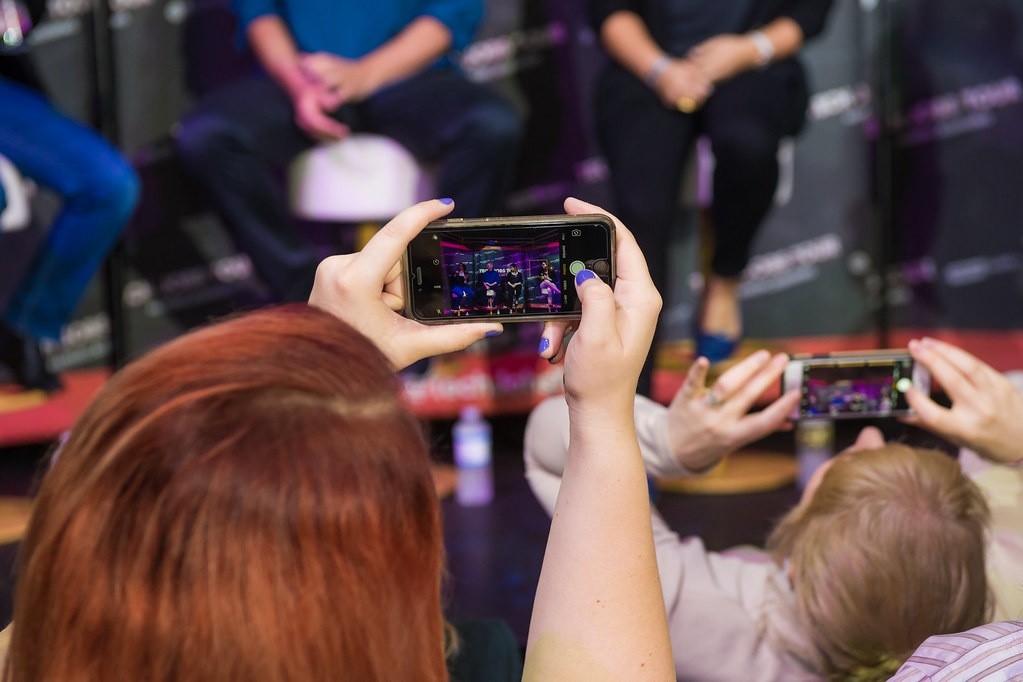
[451,262,569,316]
[0,197,678,682]
[524,337,1023,682]
[203,0,534,276]
[886,371,1023,682]
[586,0,837,363]
[0,75,142,394]
[808,384,895,414]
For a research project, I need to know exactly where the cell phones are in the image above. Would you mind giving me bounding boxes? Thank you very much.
[402,214,618,326]
[781,348,931,421]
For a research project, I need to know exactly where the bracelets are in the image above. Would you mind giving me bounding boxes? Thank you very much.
[643,52,672,87]
[359,55,387,89]
[748,28,776,68]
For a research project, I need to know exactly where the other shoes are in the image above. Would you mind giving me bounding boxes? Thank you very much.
[692,284,742,362]
[1,325,63,394]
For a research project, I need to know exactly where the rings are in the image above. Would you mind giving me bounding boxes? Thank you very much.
[708,388,729,404]
[678,98,694,111]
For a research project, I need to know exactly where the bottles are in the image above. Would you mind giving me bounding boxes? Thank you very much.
[452,404,495,509]
[794,418,834,495]
